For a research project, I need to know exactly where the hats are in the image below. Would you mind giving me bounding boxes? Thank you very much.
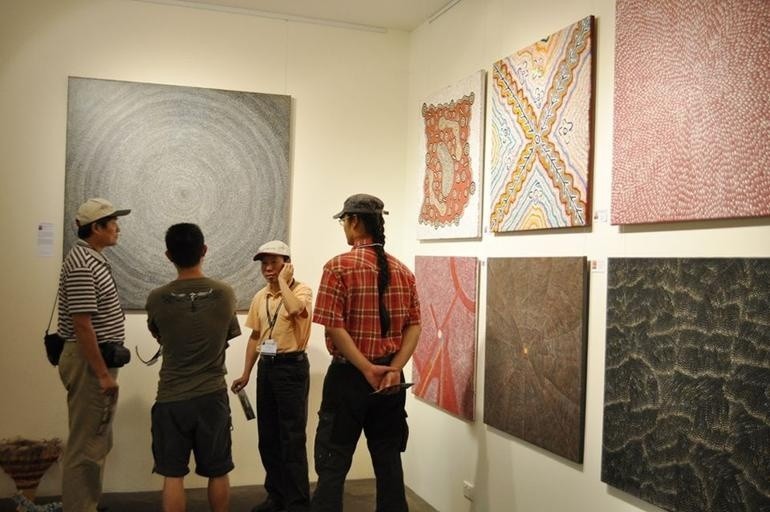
[76,198,129,226]
[253,239,289,261]
[333,195,389,219]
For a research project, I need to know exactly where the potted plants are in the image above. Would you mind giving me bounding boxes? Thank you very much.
[0,434,66,504]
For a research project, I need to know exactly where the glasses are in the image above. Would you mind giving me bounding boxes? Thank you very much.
[337,216,349,225]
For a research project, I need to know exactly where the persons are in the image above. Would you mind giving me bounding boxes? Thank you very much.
[231,240,314,511]
[307,193,422,512]
[146,222,242,512]
[56,197,132,512]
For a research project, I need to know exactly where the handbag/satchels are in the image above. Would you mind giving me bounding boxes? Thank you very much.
[44,334,64,364]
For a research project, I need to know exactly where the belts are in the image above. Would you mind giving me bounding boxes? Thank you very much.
[261,351,305,364]
